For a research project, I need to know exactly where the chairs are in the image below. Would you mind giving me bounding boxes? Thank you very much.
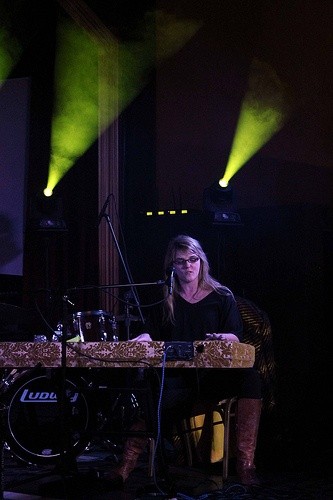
[149,296,273,478]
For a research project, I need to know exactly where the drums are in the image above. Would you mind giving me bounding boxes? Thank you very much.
[55,310,148,343]
[1,365,138,469]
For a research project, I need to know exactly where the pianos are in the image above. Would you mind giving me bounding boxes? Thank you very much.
[1,340,256,476]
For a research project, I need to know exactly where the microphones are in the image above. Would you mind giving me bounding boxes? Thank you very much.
[93,193,111,227]
[166,267,175,295]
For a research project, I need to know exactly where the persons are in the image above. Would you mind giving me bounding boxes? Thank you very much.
[111,235,263,485]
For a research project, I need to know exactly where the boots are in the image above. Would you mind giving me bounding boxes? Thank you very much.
[110,421,148,481]
[236,397,263,492]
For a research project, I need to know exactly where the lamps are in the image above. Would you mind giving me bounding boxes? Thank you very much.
[202,177,241,224]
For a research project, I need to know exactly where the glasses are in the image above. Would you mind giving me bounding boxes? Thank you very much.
[173,256,200,264]
